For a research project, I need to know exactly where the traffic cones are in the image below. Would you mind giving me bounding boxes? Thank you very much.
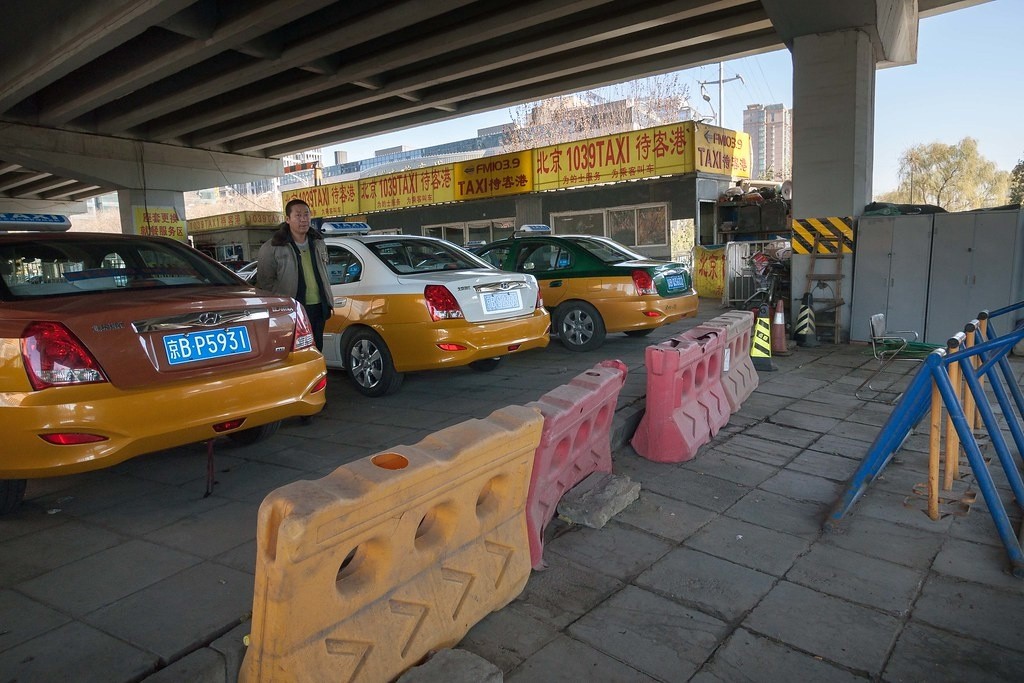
[750,303,778,372]
[772,300,792,356]
[795,293,823,347]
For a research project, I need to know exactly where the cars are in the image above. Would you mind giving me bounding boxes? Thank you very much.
[469,224,700,351]
[1,211,330,512]
[239,223,553,396]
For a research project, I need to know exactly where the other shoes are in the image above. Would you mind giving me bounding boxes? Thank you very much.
[299,416,314,426]
[318,403,328,417]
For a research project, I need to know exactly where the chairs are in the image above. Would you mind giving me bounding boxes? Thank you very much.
[854,313,947,407]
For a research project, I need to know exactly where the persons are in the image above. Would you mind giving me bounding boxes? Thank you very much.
[255,199,336,357]
